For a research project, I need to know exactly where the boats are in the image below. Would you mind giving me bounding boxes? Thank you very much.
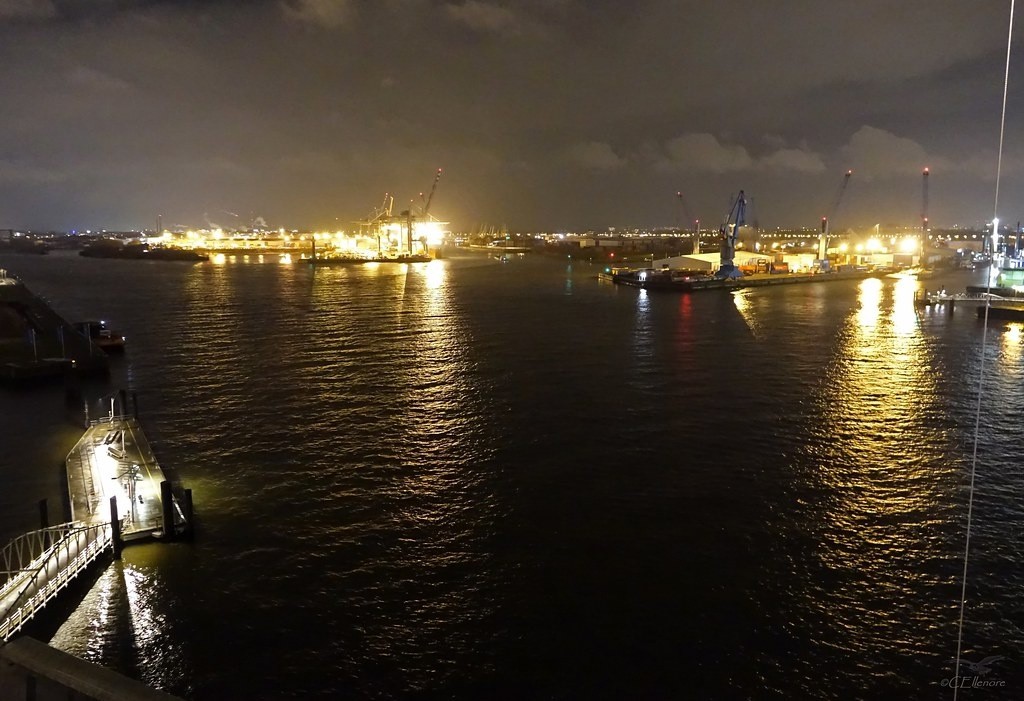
[494,254,506,262]
[909,266,943,280]
[298,193,433,263]
[72,320,126,348]
[0,269,109,389]
